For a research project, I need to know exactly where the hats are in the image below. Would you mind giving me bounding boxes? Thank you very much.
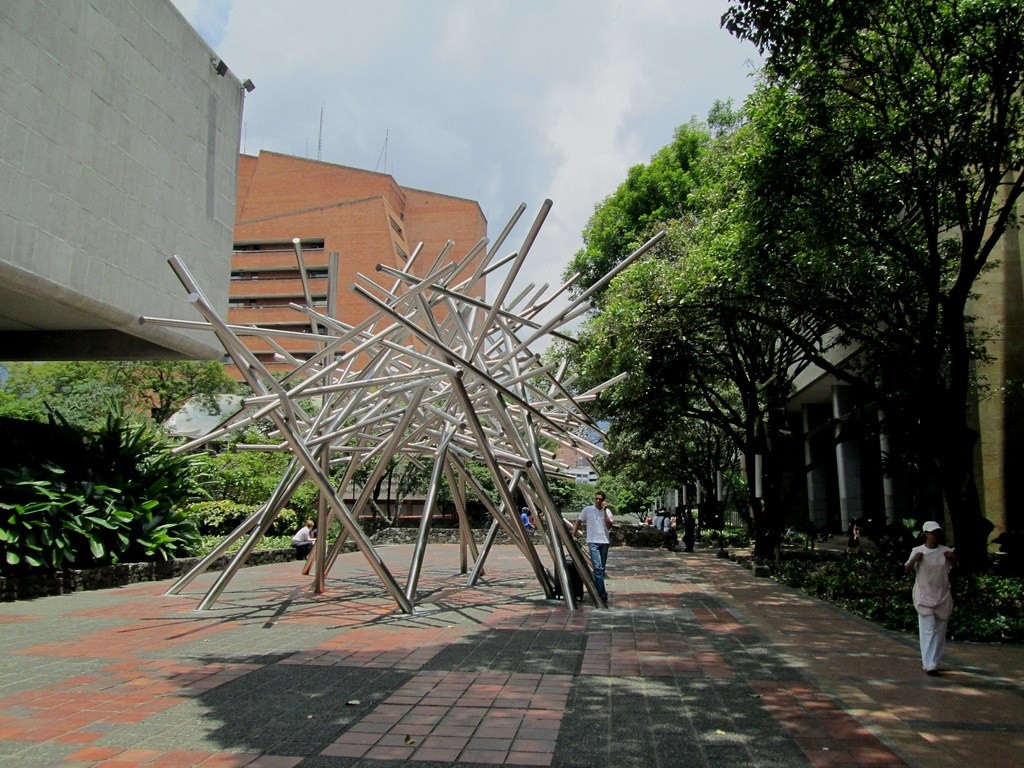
[522,507,529,512]
[922,521,943,532]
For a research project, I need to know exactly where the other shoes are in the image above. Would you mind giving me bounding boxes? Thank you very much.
[927,669,940,676]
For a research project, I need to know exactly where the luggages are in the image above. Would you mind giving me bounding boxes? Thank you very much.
[555,542,585,602]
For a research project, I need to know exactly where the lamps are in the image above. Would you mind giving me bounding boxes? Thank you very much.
[209,57,229,77]
[240,79,255,93]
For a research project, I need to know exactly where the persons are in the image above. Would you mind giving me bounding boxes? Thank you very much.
[804,521,817,550]
[846,517,860,552]
[573,491,614,602]
[291,519,328,559]
[645,510,677,532]
[520,507,547,529]
[905,522,961,676]
